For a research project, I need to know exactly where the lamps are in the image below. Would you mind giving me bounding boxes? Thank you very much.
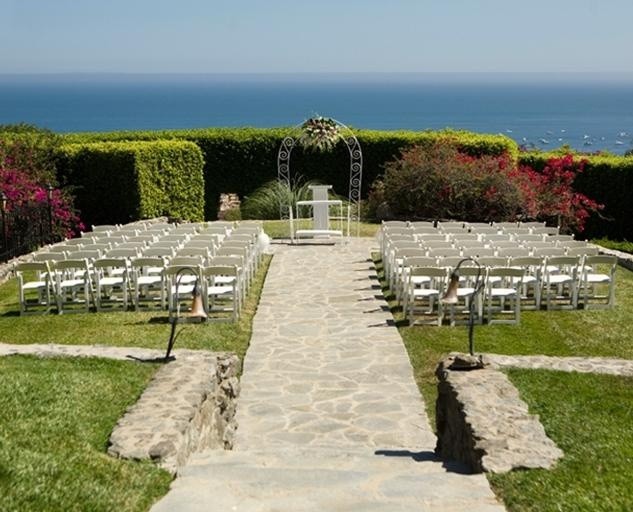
[153,266,208,364]
[437,257,487,369]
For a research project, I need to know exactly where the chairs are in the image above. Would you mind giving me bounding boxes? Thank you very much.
[376,217,619,327]
[10,216,262,321]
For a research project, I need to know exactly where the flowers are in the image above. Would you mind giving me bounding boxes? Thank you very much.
[301,114,340,154]
